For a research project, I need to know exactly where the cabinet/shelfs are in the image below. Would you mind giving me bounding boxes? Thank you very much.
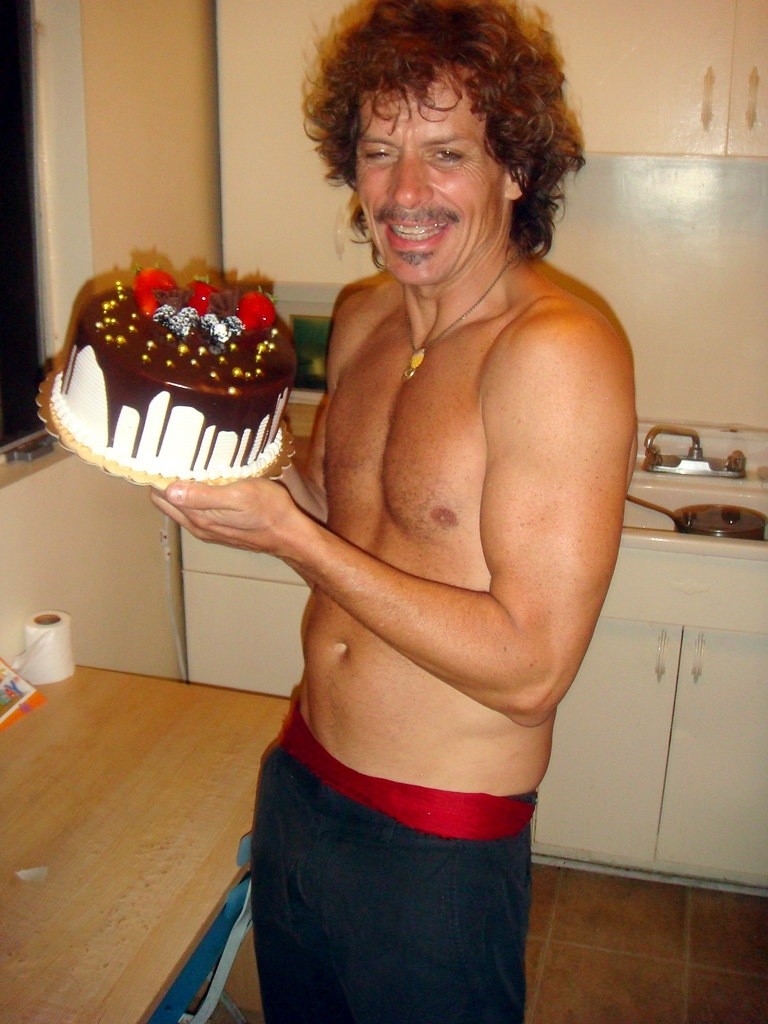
[178,522,768,897]
[522,0,768,158]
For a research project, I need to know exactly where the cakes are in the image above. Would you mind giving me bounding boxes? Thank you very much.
[49,264,297,482]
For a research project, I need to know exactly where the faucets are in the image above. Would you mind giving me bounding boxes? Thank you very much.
[641,423,747,479]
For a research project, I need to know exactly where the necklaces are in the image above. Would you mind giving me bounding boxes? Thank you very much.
[402,254,519,382]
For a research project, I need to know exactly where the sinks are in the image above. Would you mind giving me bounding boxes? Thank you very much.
[617,470,768,562]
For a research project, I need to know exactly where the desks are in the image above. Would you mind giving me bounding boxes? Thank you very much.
[0,665,292,1024]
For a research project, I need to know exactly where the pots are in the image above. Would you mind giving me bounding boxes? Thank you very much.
[626,493,768,540]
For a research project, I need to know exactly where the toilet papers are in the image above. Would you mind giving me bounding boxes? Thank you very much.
[9,609,76,687]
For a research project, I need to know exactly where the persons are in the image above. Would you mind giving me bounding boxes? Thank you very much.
[149,0,636,1024]
